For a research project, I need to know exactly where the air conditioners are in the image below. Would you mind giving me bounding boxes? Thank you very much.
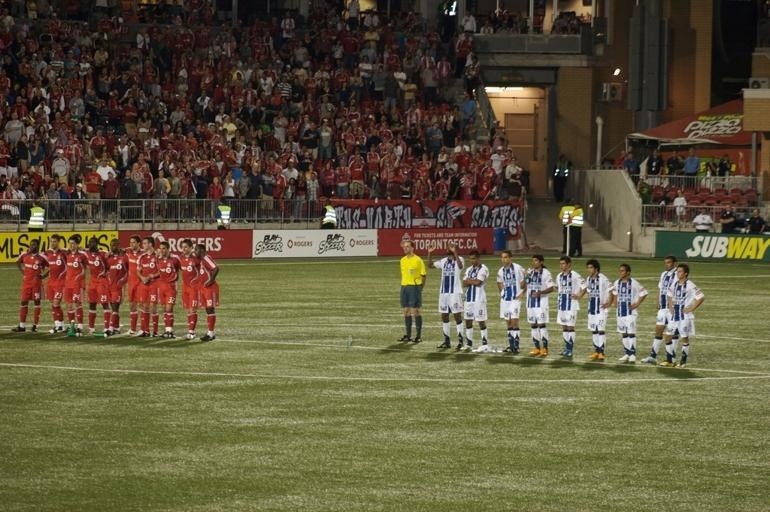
[597,83,622,102]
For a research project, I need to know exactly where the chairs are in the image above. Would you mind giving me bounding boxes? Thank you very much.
[639,184,760,223]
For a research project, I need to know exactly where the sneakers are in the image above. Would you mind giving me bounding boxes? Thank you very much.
[12,326,26,332]
[436,342,452,348]
[618,353,630,361]
[628,353,636,362]
[31,324,218,342]
[659,360,675,367]
[674,362,686,369]
[412,336,422,342]
[640,356,657,363]
[453,343,607,360]
[396,335,412,341]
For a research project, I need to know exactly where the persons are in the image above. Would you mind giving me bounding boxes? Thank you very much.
[609,145,765,237]
[1,1,607,232]
[396,241,704,368]
[554,198,586,260]
[13,235,220,342]
